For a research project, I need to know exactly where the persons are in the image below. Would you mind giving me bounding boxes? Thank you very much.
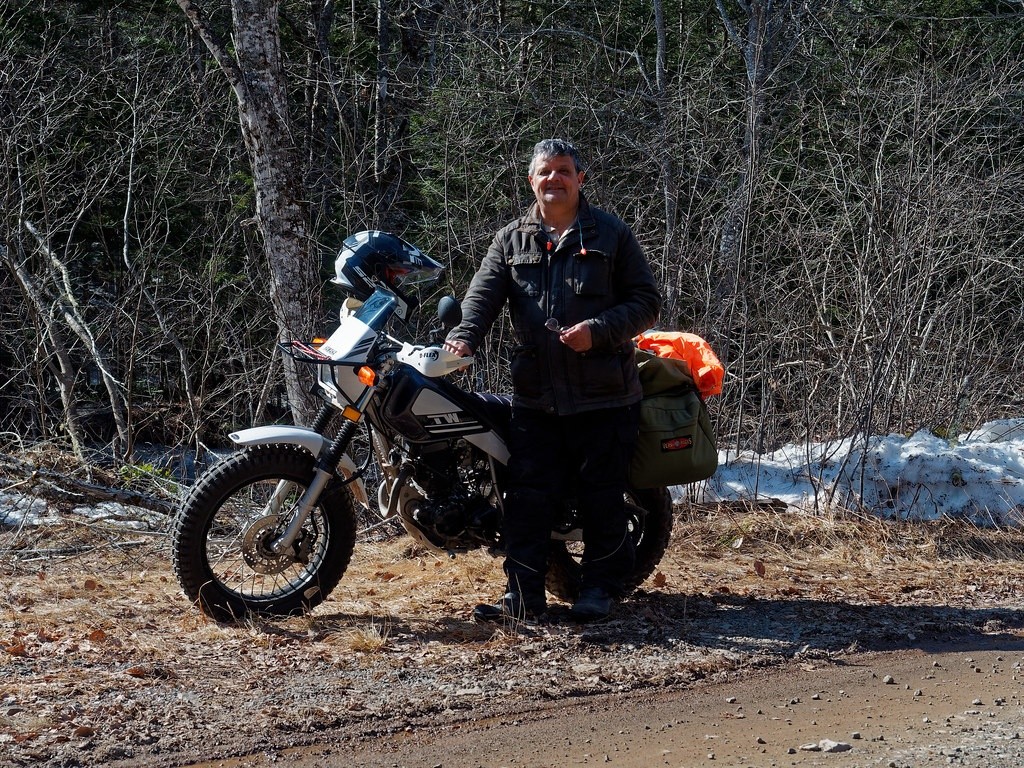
[444,140,662,626]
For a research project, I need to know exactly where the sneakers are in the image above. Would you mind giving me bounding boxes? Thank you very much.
[572,569,613,619]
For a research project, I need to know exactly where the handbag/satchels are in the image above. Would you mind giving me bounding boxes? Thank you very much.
[629,347,718,489]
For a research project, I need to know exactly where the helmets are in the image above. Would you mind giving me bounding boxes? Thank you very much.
[329,229,447,326]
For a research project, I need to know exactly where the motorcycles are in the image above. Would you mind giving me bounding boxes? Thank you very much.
[170,230,696,624]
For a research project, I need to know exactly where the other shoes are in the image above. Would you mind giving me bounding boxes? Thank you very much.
[474,567,547,626]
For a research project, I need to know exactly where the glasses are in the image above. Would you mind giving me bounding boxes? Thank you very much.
[545,318,570,336]
